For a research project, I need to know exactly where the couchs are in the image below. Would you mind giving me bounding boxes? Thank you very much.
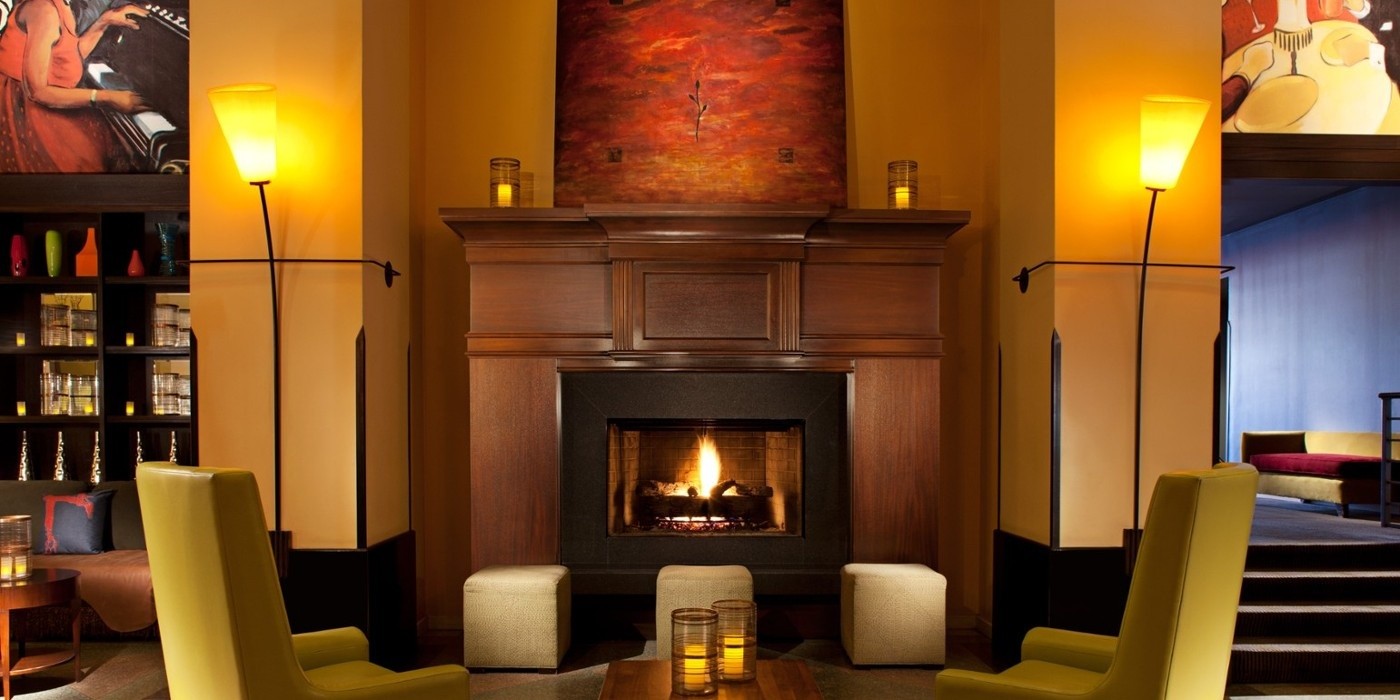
[0,479,162,654]
[1240,430,1400,518]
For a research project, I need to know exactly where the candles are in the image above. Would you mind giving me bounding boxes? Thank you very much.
[717,628,757,682]
[887,184,916,211]
[674,637,714,692]
[491,181,514,207]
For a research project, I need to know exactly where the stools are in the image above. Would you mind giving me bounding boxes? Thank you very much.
[650,566,754,651]
[458,564,571,673]
[835,563,947,665]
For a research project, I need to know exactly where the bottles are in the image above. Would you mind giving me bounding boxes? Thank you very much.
[18,430,103,485]
[128,250,145,277]
[134,431,178,483]
[152,223,179,276]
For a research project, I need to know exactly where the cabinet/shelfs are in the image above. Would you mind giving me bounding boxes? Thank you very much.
[0,170,209,487]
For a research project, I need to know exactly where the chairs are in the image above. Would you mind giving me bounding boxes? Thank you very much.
[131,455,472,700]
[934,458,1260,700]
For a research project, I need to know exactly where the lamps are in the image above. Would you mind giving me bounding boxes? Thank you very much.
[201,79,308,553]
[1103,88,1224,556]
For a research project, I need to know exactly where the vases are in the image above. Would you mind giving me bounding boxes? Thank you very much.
[128,246,144,277]
[152,218,182,275]
[5,230,31,278]
[43,228,62,277]
[74,224,99,277]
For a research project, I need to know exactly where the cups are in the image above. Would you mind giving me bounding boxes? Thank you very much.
[711,598,756,683]
[41,303,98,346]
[150,304,190,347]
[489,157,521,210]
[39,372,100,416]
[887,159,920,208]
[671,608,718,695]
[153,372,191,416]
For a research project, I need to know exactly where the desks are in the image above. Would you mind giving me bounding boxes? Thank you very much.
[0,562,84,700]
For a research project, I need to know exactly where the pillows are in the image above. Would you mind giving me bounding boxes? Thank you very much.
[34,491,112,554]
